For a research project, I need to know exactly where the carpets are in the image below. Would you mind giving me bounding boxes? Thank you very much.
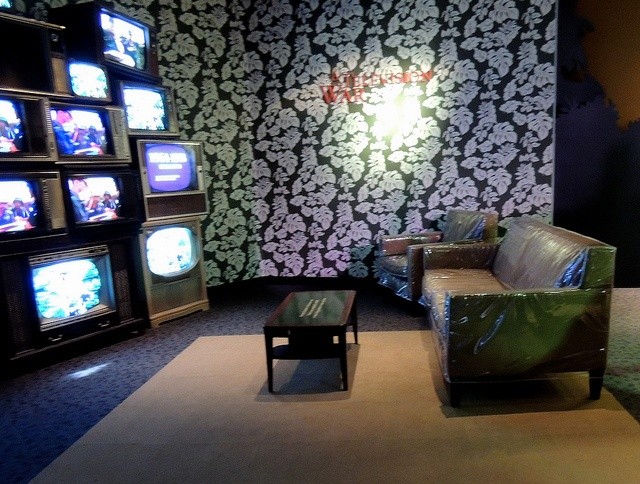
[29,329,639,484]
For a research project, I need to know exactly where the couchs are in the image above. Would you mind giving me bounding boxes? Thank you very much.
[379,210,498,319]
[424,216,617,407]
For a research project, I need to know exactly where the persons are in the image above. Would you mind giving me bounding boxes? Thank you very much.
[51,110,79,154]
[103,192,116,210]
[84,125,103,146]
[0,201,15,226]
[69,177,102,222]
[0,117,14,141]
[8,195,27,220]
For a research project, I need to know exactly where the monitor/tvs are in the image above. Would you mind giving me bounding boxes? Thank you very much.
[0,170,68,252]
[67,58,112,105]
[49,1,158,78]
[131,220,210,329]
[20,244,119,348]
[0,93,59,162]
[50,100,133,168]
[114,78,180,138]
[60,169,145,242]
[0,1,16,14]
[134,139,210,222]
